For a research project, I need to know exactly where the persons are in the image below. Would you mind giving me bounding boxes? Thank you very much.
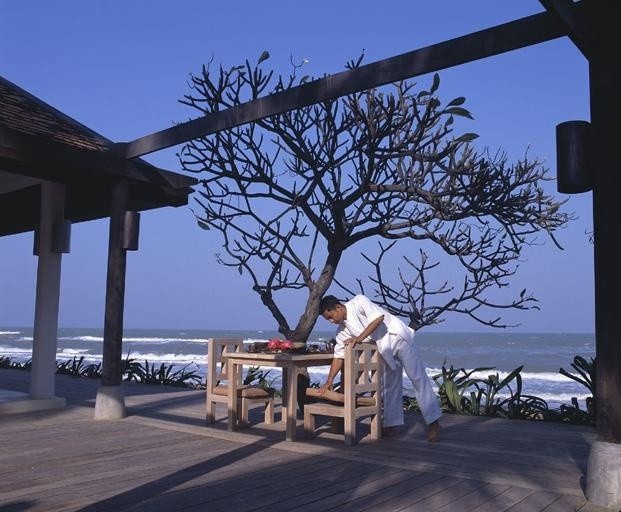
[315,292,445,442]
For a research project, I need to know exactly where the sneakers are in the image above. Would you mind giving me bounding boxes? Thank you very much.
[364,427,396,437]
[427,420,439,442]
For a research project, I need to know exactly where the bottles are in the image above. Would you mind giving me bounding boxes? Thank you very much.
[318,338,327,352]
[329,338,337,354]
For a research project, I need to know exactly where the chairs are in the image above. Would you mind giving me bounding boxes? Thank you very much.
[205,338,383,446]
